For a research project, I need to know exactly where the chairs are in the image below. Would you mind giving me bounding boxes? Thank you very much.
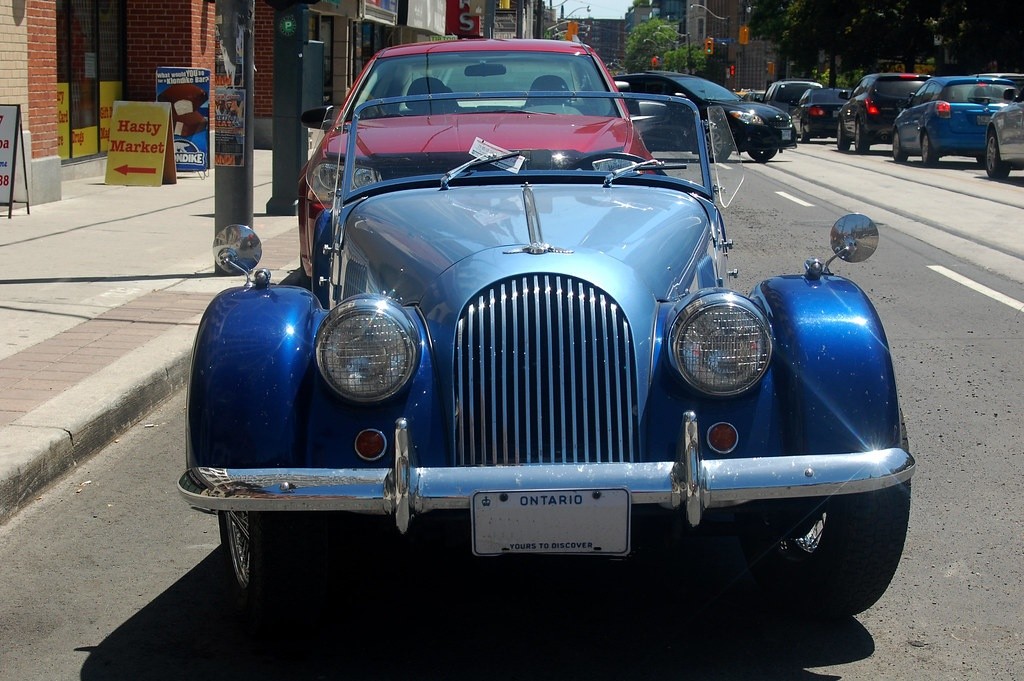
[525,75,573,107]
[405,77,460,112]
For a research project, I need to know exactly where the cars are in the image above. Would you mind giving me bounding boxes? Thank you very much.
[177,37,917,640]
[733,72,1024,181]
[607,70,798,163]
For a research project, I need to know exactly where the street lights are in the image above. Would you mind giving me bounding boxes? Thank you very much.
[546,0,592,45]
[690,3,729,85]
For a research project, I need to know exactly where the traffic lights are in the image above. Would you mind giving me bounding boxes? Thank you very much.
[650,57,657,67]
[704,37,714,55]
[729,64,737,75]
[739,27,751,45]
